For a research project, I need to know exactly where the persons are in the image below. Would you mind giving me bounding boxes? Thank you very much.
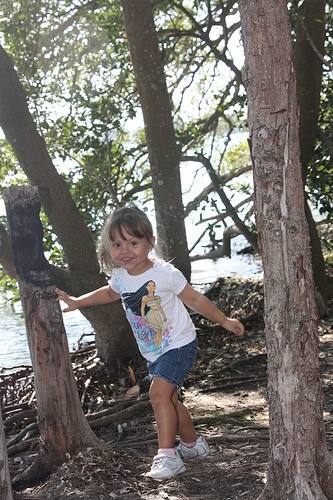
[55,206,244,483]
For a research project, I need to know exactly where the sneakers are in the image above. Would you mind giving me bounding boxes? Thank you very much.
[144,450,187,481]
[176,436,210,462]
